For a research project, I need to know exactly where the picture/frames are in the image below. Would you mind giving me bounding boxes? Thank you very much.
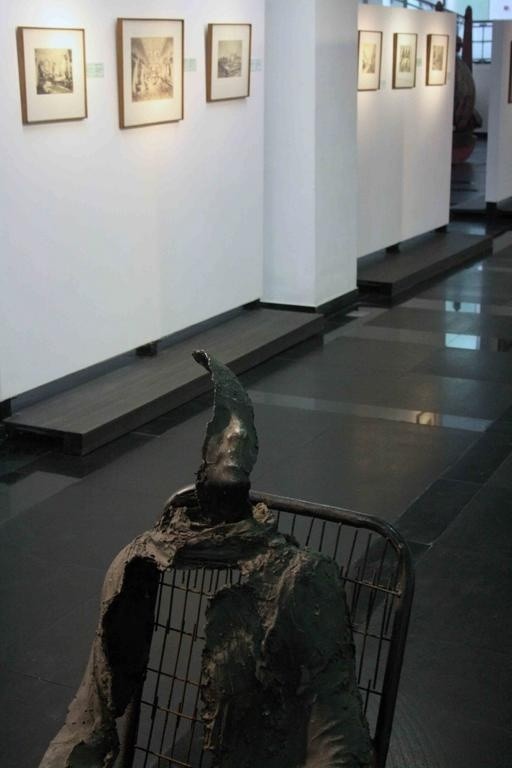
[116,17,185,129]
[392,32,417,89]
[357,29,384,93]
[425,34,450,86]
[15,25,90,126]
[207,22,253,102]
